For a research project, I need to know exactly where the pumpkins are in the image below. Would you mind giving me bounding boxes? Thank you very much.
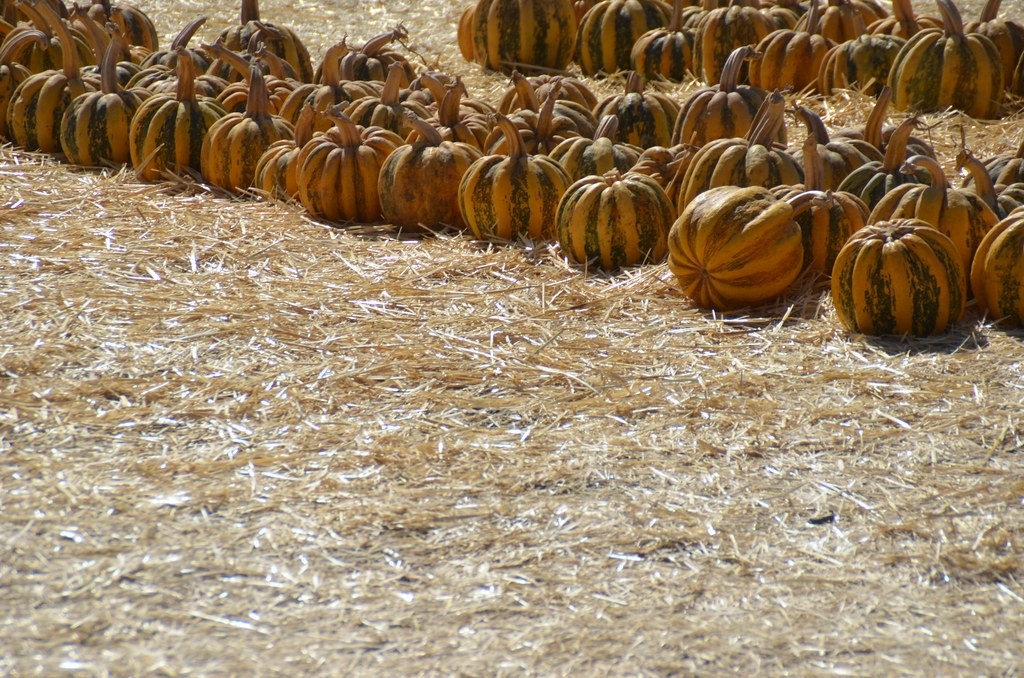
[1,0,1024,343]
